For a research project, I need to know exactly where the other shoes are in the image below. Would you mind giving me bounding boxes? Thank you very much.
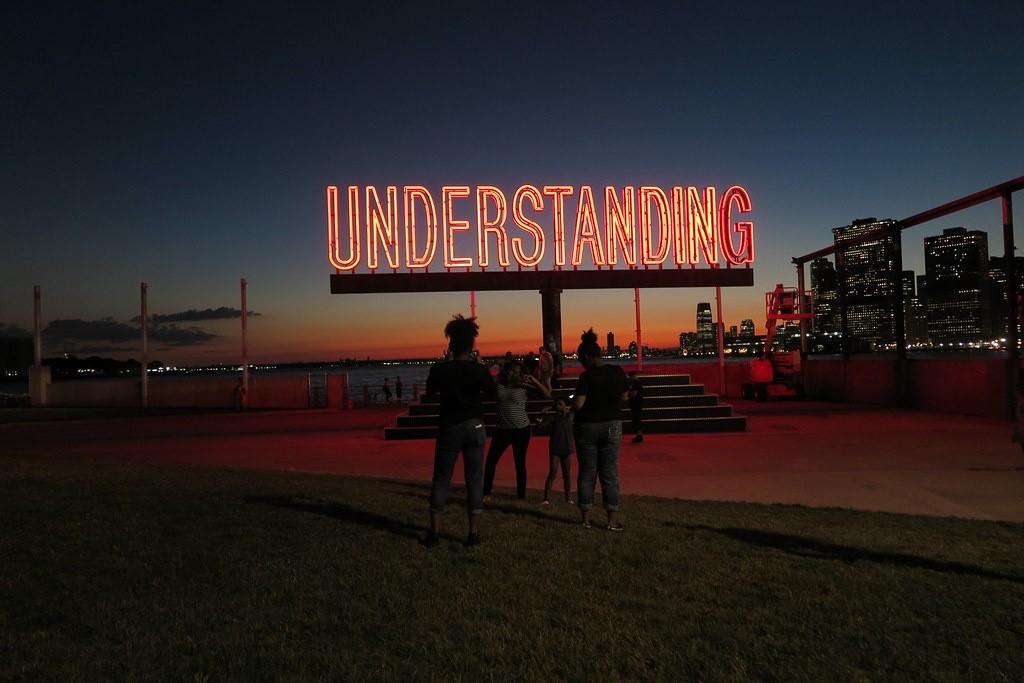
[542,501,549,505]
[607,521,623,531]
[420,534,439,548]
[582,519,593,530]
[632,435,642,442]
[469,532,482,544]
[567,500,574,504]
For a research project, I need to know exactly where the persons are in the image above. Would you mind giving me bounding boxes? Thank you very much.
[382,378,392,402]
[534,399,575,507]
[426,316,497,547]
[571,332,626,530]
[474,360,552,503]
[395,375,405,402]
[504,341,562,392]
[628,371,645,444]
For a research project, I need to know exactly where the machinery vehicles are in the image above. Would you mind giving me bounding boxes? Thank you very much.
[741,282,816,403]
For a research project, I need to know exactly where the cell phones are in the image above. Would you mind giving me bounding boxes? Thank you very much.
[469,351,478,361]
[569,394,574,399]
